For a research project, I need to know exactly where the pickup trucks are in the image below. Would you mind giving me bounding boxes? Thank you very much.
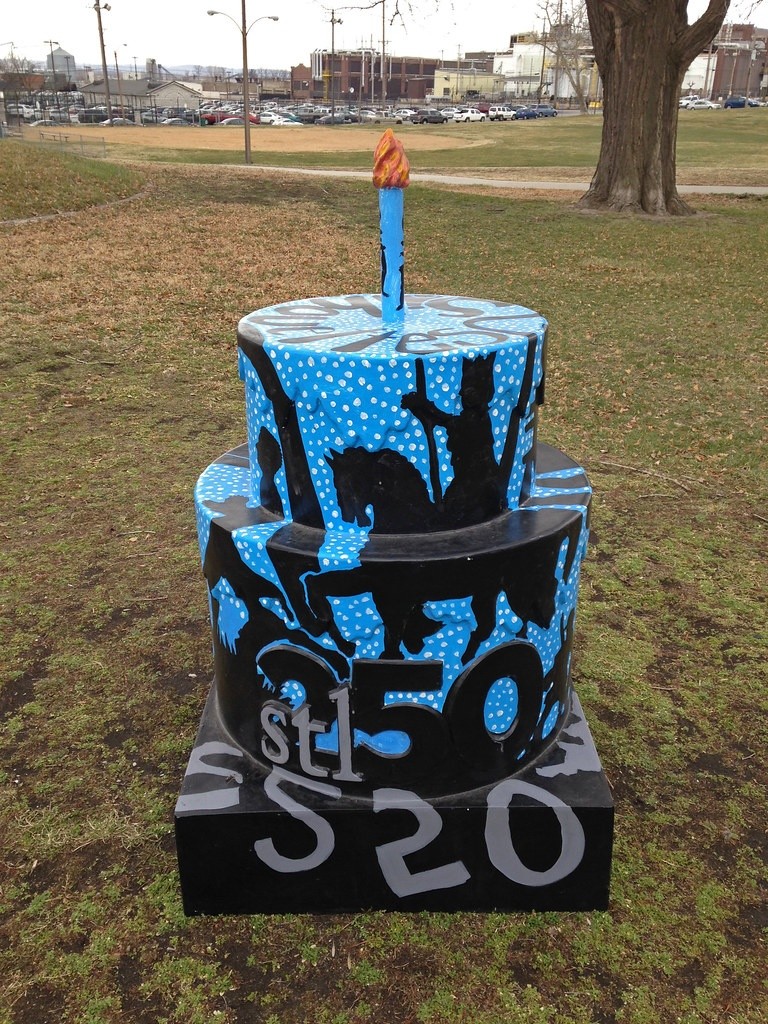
[361,111,403,125]
[462,90,486,101]
[453,108,486,123]
[410,110,449,124]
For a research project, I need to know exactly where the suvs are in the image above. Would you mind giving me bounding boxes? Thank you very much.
[489,107,516,121]
[531,105,558,118]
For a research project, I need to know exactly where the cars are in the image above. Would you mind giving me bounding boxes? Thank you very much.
[680,94,765,110]
[214,118,257,125]
[100,117,147,126]
[315,116,351,124]
[272,118,302,126]
[161,117,198,126]
[6,101,529,123]
[30,120,70,126]
[515,108,538,120]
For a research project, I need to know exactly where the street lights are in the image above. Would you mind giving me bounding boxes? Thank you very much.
[96,4,112,127]
[207,10,278,163]
[44,41,61,119]
[114,44,127,125]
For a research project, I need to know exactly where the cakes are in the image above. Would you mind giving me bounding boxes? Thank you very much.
[194,294,591,800]
